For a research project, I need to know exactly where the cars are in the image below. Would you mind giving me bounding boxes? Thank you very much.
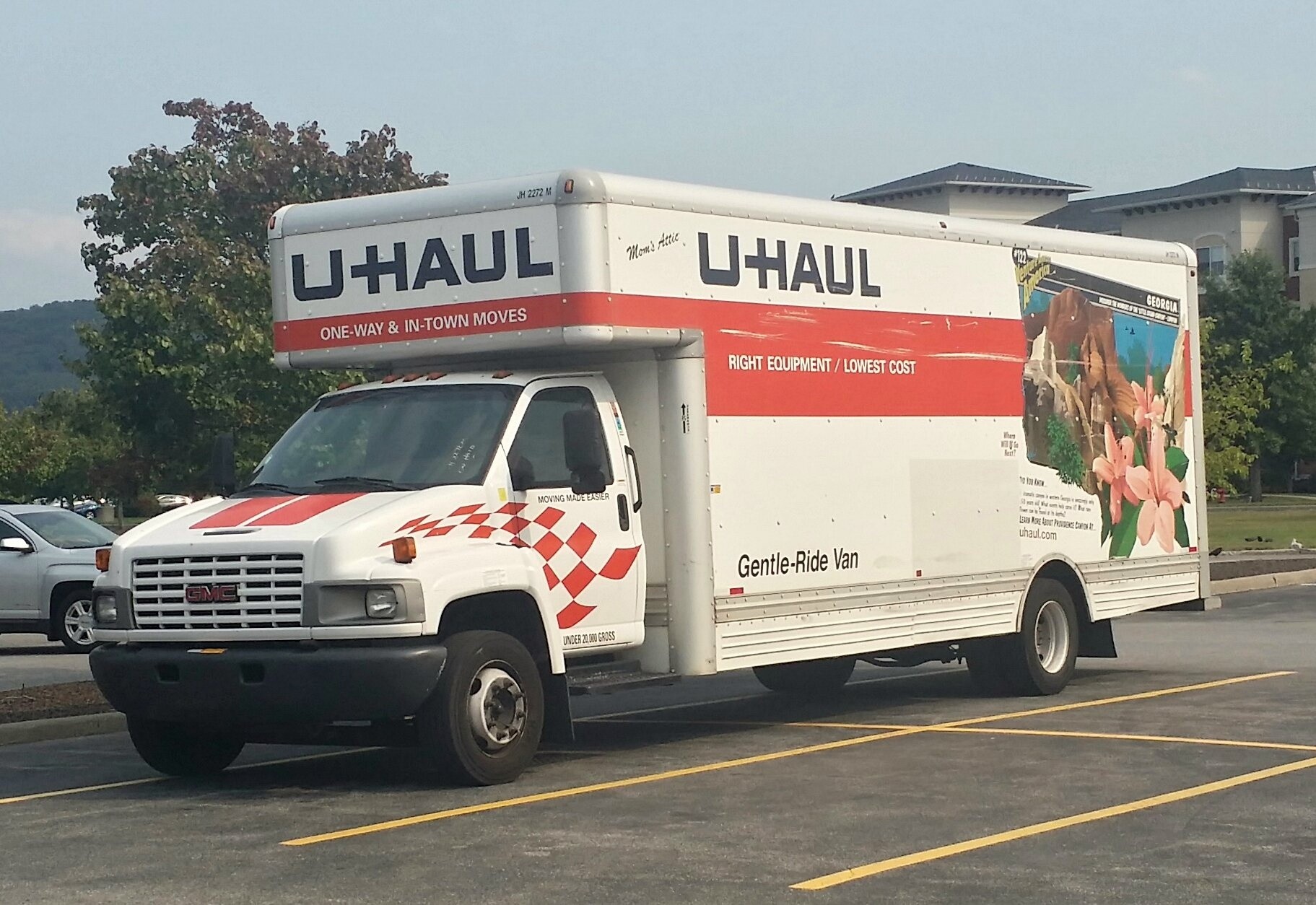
[49,495,117,521]
[152,493,192,513]
[0,502,120,656]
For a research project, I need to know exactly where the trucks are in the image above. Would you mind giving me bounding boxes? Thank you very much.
[83,161,1224,791]
